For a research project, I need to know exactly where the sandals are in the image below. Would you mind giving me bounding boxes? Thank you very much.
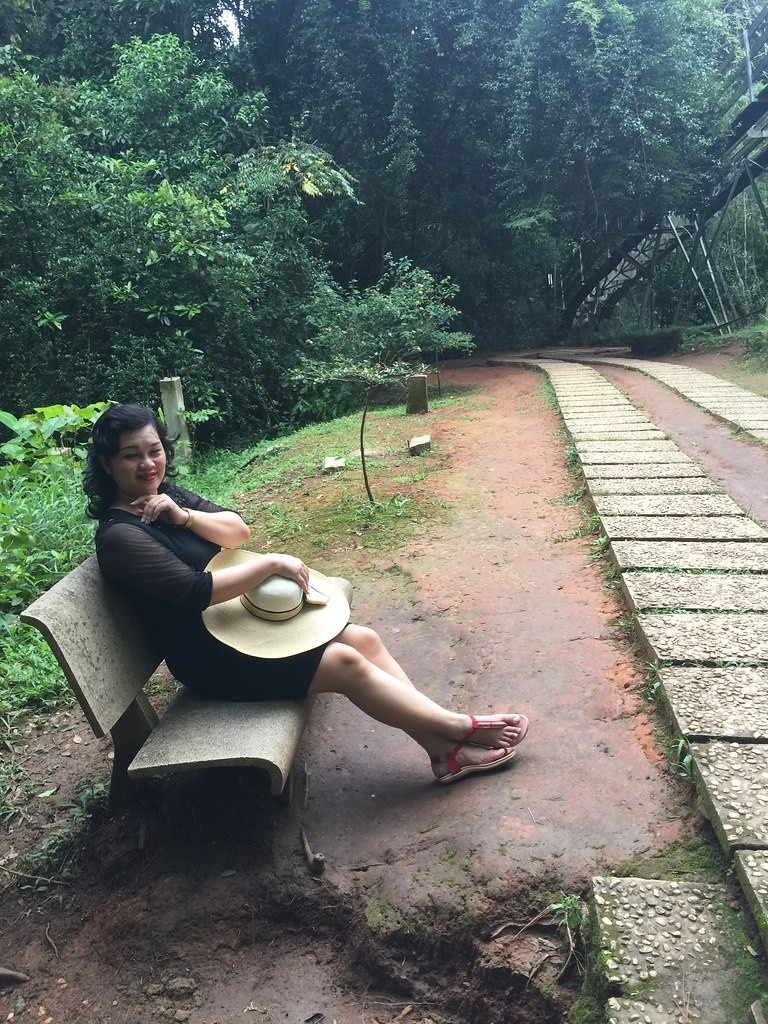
[464,714,529,748]
[431,742,517,784]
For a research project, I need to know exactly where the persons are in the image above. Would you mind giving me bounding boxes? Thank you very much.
[80,405,530,787]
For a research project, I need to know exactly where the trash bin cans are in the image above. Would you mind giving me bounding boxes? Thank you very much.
[406,375,429,414]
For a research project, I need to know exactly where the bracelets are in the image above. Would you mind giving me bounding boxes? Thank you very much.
[180,508,194,529]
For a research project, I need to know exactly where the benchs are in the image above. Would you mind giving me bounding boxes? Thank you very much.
[20,551,316,811]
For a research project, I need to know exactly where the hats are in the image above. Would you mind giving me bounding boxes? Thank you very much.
[201,549,350,659]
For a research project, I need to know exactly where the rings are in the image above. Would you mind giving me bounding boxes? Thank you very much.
[156,499,161,504]
